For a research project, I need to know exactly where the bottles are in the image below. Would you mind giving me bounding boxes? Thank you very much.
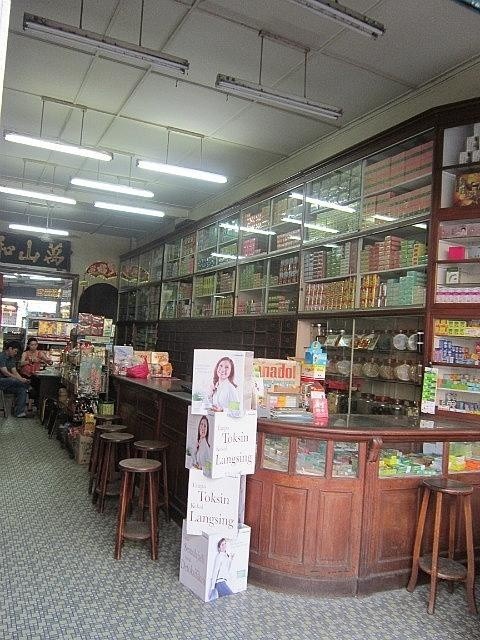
[67,397,97,423]
[288,296,297,311]
[329,392,419,417]
[310,323,424,353]
[324,354,423,385]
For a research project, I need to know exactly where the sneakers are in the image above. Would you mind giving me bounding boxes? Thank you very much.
[16,411,28,418]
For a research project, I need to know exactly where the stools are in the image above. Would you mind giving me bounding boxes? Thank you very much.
[405,476,478,616]
[86,414,169,561]
[0,388,9,418]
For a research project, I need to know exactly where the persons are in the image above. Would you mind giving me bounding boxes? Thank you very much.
[193,415,210,470]
[0,340,34,418]
[203,357,239,413]
[19,338,49,412]
[208,538,234,600]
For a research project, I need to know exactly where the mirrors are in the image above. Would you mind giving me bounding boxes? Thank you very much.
[0,265,79,330]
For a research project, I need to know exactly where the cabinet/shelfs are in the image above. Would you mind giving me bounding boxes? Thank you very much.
[23,316,80,364]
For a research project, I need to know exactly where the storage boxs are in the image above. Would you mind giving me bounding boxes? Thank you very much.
[178,348,257,603]
[75,434,93,465]
